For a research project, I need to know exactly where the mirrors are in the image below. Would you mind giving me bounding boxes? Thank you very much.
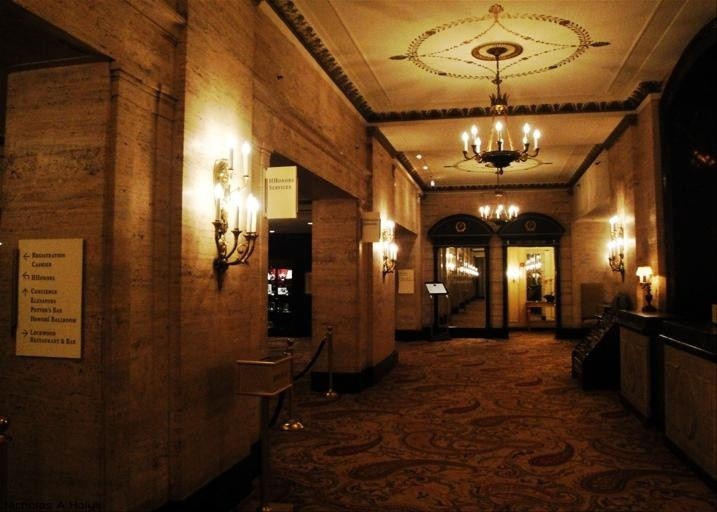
[433,244,489,330]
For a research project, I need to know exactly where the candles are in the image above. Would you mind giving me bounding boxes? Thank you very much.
[214,132,258,236]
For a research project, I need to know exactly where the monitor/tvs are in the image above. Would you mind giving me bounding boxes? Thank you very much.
[267,269,295,314]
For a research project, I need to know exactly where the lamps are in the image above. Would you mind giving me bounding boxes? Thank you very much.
[461,47,543,225]
